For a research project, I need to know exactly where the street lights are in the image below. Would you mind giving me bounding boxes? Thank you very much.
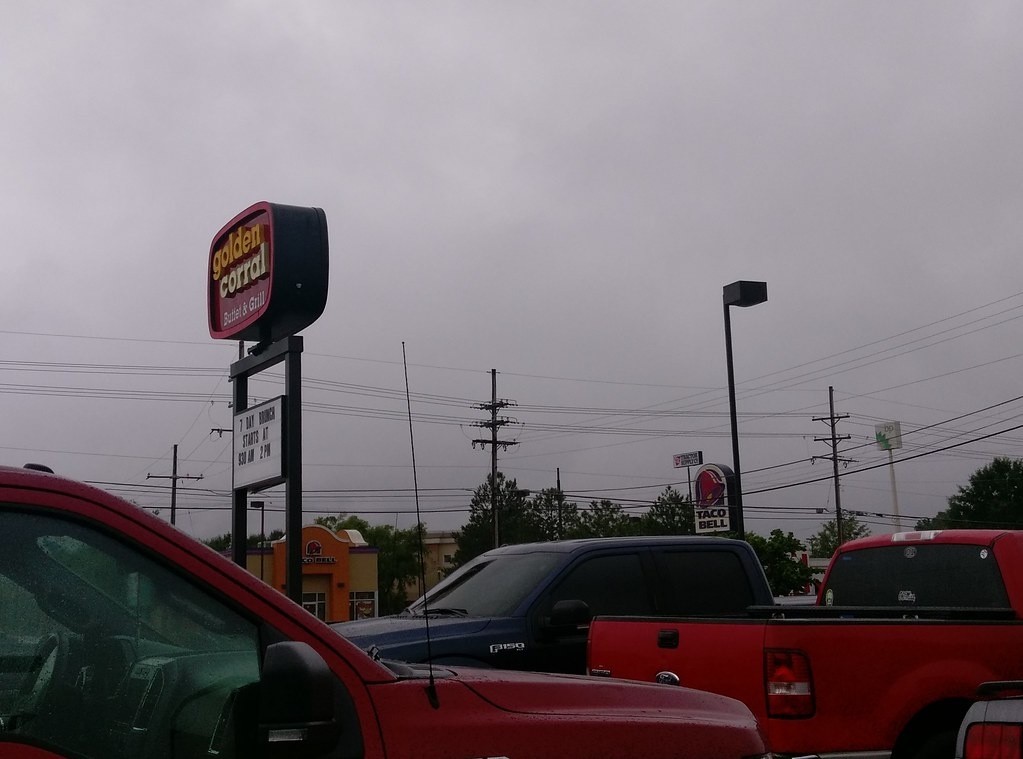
[722,280,769,537]
[251,501,263,581]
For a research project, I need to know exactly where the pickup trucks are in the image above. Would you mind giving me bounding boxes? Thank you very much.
[322,537,1023,759]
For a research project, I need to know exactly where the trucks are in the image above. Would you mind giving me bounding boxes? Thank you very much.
[819,525,1022,622]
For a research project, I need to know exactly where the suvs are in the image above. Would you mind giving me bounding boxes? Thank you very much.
[0,462,766,759]
